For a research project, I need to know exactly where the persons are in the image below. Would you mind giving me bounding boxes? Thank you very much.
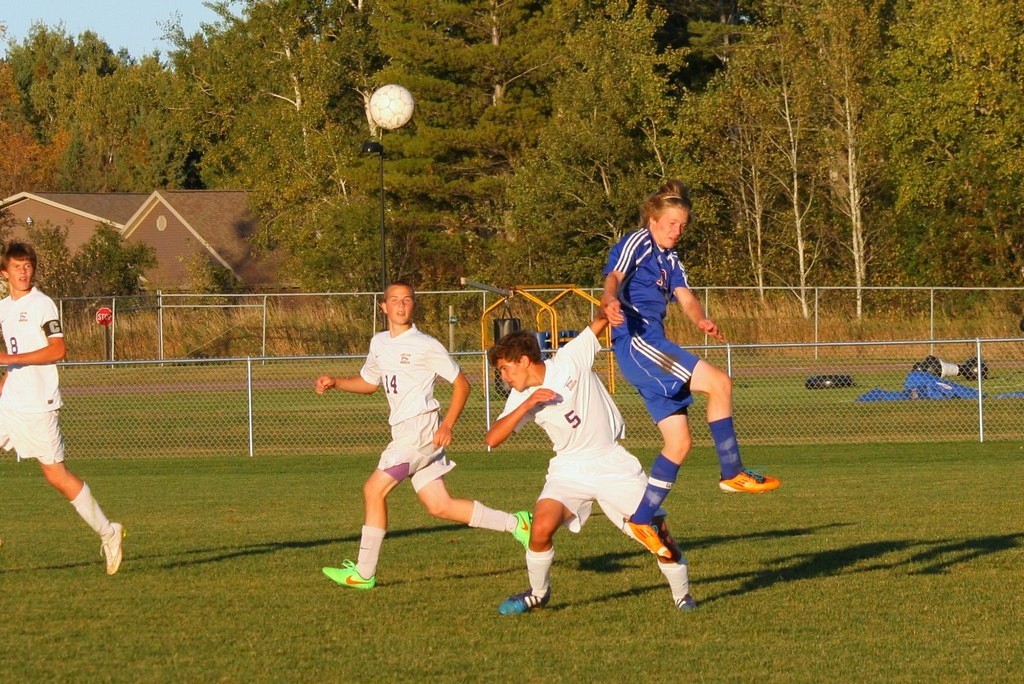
[316,281,533,591]
[603,180,781,559]
[487,297,696,614]
[0,245,126,576]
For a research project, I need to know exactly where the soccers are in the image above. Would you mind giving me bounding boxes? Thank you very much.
[368,82,416,131]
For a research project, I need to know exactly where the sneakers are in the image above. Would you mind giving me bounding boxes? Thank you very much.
[513,511,533,550]
[624,510,682,564]
[674,594,697,611]
[499,587,551,615]
[322,559,375,589]
[100,523,126,575]
[719,468,781,494]
